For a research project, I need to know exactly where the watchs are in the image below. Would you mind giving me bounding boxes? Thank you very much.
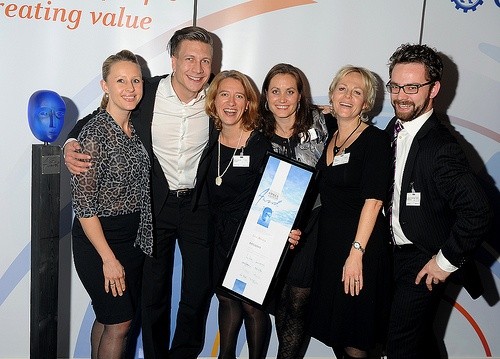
[352,241,365,253]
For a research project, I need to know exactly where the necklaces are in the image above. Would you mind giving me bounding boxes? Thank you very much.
[215,126,244,186]
[333,119,362,158]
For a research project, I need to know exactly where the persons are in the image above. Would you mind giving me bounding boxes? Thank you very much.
[316,64,391,359]
[71,50,153,353]
[255,63,338,359]
[206,70,275,359]
[62,27,217,359]
[370,43,491,359]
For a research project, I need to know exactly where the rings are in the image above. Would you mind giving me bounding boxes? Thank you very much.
[110,283,115,285]
[355,280,359,282]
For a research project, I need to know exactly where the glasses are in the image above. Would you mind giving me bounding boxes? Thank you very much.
[386,80,432,94]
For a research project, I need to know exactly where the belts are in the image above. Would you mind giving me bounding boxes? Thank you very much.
[169,188,194,200]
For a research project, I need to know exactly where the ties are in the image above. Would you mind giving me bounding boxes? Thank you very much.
[386,121,404,246]
